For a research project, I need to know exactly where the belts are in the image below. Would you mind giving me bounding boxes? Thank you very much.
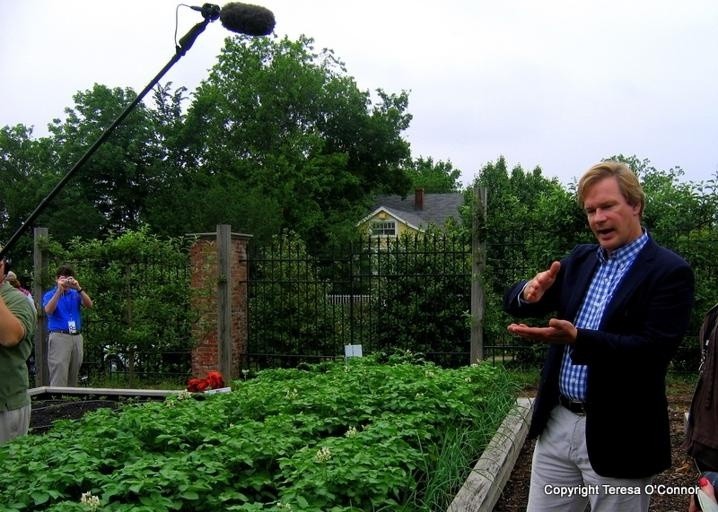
[561,397,587,416]
[52,330,82,336]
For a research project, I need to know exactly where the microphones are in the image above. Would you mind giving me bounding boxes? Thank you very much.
[191,3,275,37]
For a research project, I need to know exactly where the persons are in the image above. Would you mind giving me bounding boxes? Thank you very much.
[43,265,93,388]
[503,163,694,512]
[0,242,38,446]
[685,301,716,512]
[7,269,35,308]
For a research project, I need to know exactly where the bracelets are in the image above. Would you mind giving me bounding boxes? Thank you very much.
[78,288,84,293]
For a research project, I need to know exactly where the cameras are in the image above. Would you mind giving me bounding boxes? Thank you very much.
[64,279,72,287]
